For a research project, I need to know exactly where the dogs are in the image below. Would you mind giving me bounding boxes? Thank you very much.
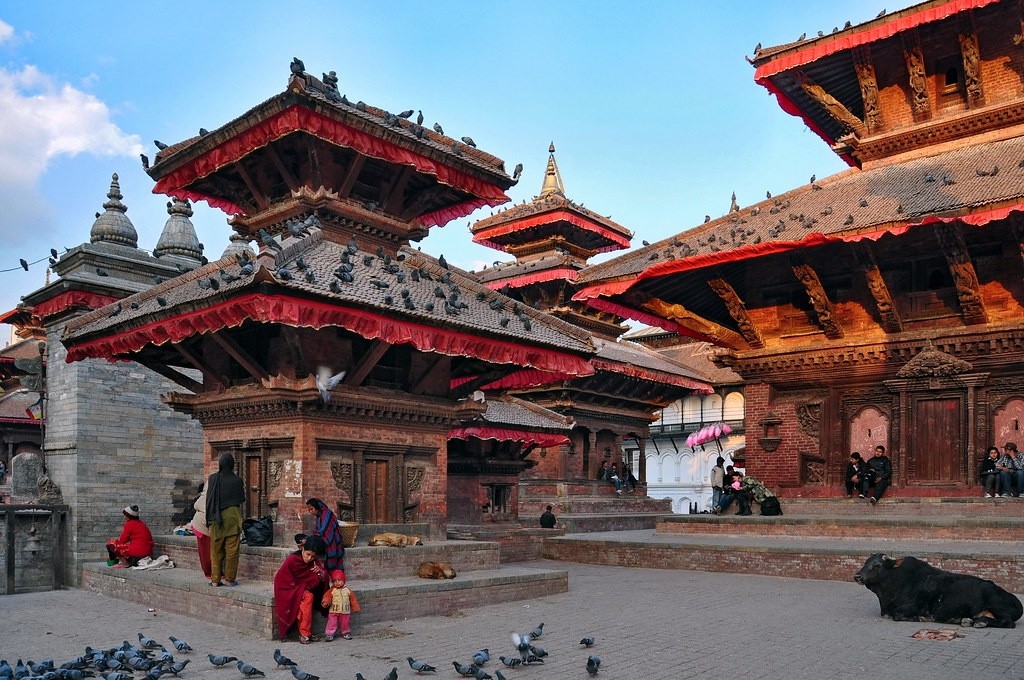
[367,530,424,548]
[416,559,457,580]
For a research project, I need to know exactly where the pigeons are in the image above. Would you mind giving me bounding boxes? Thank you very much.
[585,655,602,678]
[638,159,1024,264]
[205,653,239,667]
[354,672,367,680]
[450,620,551,680]
[753,8,887,56]
[19,257,30,272]
[578,636,595,649]
[93,189,613,335]
[316,371,346,402]
[235,660,266,680]
[406,656,437,675]
[48,248,58,265]
[382,666,399,680]
[273,648,299,671]
[0,631,194,680]
[289,665,320,680]
[136,124,214,212]
[512,162,524,179]
[289,53,476,158]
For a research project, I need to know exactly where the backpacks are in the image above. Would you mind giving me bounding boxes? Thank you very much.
[759,495,784,516]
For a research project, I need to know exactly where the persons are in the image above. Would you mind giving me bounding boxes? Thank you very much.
[858,445,891,504]
[977,447,1001,498]
[106,505,155,568]
[306,498,345,574]
[205,453,245,586]
[294,534,325,568]
[995,442,1024,498]
[321,570,361,641]
[191,480,224,580]
[540,505,556,529]
[599,460,638,495]
[273,536,329,644]
[731,471,783,515]
[717,465,744,516]
[845,452,866,498]
[710,457,725,512]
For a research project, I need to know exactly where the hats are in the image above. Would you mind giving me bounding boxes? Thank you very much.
[612,463,617,466]
[331,569,346,583]
[123,505,139,519]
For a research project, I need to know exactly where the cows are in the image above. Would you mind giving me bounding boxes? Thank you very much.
[851,548,1024,631]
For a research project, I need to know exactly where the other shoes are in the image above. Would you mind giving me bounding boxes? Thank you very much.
[735,510,743,515]
[342,634,353,640]
[299,635,310,644]
[226,581,238,586]
[113,563,130,567]
[212,582,223,587]
[325,635,334,641]
[713,506,721,516]
[309,635,319,642]
[741,510,752,516]
